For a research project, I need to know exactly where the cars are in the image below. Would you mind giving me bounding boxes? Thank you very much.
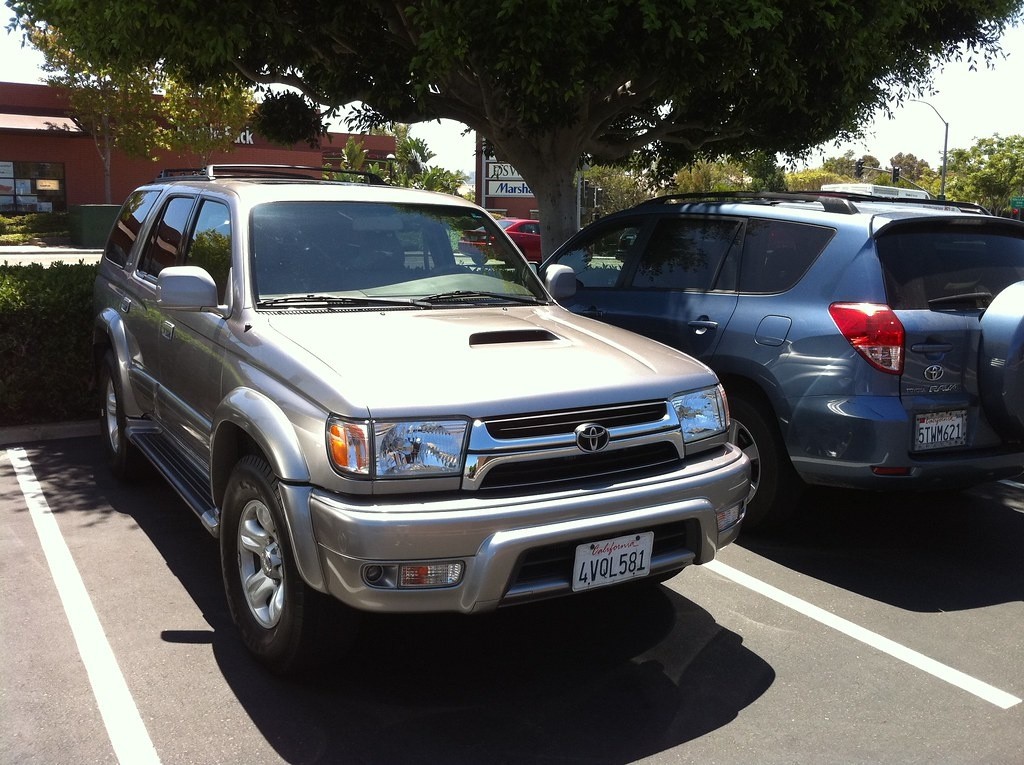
[458,218,594,269]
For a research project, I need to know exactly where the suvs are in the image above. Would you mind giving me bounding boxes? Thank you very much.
[614,225,644,260]
[94,160,751,668]
[505,190,1024,530]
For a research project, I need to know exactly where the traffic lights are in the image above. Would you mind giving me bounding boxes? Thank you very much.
[853,160,865,178]
[892,166,900,183]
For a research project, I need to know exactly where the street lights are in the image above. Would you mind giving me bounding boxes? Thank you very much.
[911,97,950,202]
